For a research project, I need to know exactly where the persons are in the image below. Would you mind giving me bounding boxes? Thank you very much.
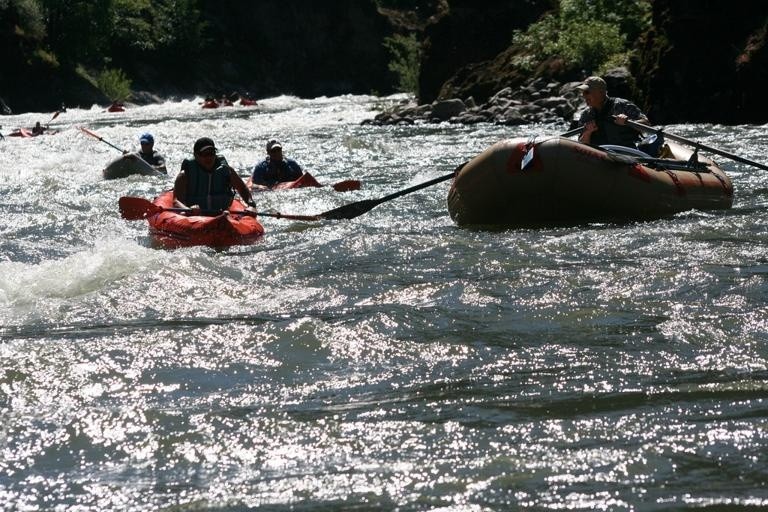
[576,76,649,151]
[123,133,167,174]
[33,122,49,136]
[173,138,258,217]
[205,90,254,104]
[252,140,303,191]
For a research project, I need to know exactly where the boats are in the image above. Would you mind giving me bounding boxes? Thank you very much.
[148,191,265,249]
[446,133,737,232]
[245,171,325,189]
[222,98,233,107]
[238,95,258,105]
[19,126,60,138]
[103,153,169,180]
[107,104,125,113]
[202,99,220,109]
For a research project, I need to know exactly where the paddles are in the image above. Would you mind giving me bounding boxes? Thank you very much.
[320,125,588,219]
[315,180,362,192]
[81,126,125,152]
[118,197,319,220]
[46,111,59,127]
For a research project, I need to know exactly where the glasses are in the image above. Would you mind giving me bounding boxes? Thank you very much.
[198,148,217,158]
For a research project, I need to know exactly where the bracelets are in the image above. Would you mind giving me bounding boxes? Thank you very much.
[249,201,256,208]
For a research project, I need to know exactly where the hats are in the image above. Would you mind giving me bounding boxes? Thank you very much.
[194,136,216,156]
[577,77,606,92]
[266,138,282,151]
[139,133,155,144]
